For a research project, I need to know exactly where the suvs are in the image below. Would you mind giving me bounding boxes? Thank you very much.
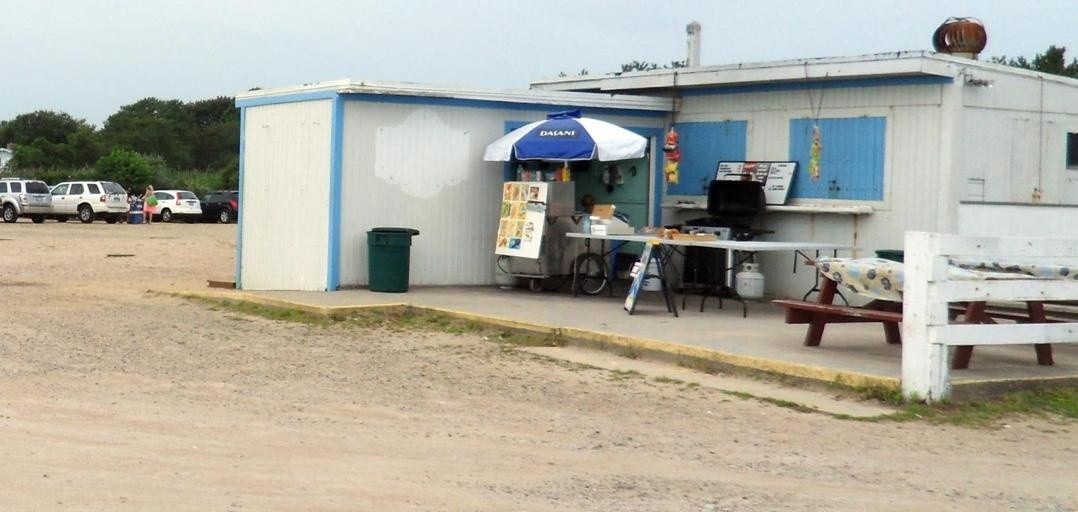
[184,190,239,225]
[0,178,54,224]
[140,190,203,223]
[49,181,131,224]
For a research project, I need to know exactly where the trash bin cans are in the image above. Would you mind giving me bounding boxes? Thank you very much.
[367,227,419,292]
[875,250,904,264]
[129,201,143,224]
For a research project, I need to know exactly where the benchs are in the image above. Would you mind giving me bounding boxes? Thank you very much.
[773,300,1003,370]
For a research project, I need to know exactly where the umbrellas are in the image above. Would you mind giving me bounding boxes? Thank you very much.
[483,113,649,168]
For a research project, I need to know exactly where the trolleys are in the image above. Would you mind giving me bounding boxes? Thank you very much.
[499,173,627,296]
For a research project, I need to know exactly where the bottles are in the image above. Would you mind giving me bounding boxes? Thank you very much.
[516,164,570,182]
[583,216,600,235]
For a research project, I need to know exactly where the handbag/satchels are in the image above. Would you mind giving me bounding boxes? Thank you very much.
[147,195,158,207]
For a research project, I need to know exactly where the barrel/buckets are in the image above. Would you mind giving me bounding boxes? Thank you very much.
[368,232,412,292]
[642,259,662,291]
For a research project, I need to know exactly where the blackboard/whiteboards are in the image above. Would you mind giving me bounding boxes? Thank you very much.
[624,243,653,311]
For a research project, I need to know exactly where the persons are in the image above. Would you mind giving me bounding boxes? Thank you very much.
[120,186,132,224]
[580,193,596,214]
[141,185,155,224]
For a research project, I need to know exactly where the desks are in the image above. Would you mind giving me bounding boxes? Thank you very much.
[771,254,1078,371]
[564,231,862,316]
